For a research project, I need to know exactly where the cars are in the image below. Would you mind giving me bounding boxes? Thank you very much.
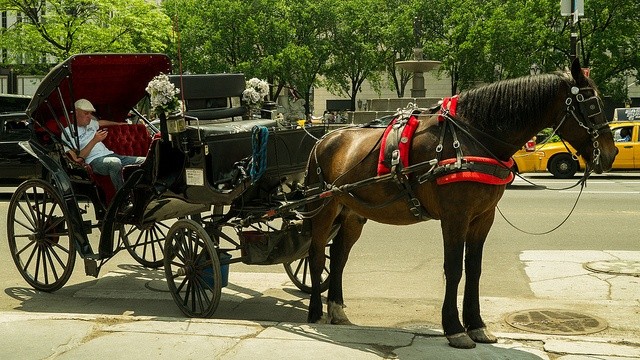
[504,135,538,188]
[536,121,640,180]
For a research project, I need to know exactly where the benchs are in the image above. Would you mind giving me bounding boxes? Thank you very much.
[170,73,276,148]
[68,123,151,220]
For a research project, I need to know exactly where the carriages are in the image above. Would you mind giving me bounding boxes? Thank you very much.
[5,51,620,350]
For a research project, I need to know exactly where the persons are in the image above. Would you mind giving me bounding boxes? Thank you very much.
[61,99,148,211]
[617,128,630,141]
[323,110,348,123]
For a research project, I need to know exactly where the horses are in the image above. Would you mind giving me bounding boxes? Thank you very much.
[302,57,619,349]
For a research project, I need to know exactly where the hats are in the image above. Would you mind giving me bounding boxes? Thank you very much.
[75,99,96,112]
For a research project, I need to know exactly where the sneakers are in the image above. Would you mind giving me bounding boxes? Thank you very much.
[116,199,134,216]
